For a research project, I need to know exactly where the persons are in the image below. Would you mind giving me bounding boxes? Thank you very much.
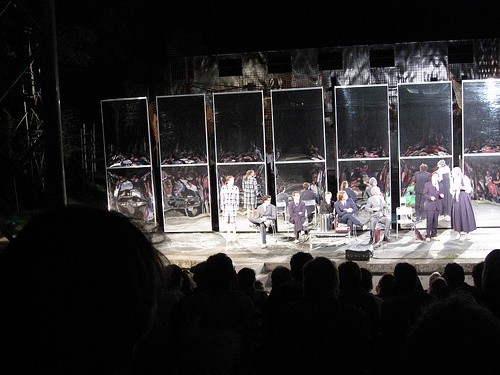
[218,174,241,242]
[247,194,277,249]
[0,164,500,375]
[333,190,367,237]
[204,137,500,207]
[422,171,445,238]
[289,189,310,243]
[448,166,478,235]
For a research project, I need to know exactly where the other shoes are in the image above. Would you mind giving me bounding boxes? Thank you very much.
[465,233,471,240]
[431,237,440,241]
[294,239,300,244]
[261,244,267,249]
[383,237,391,242]
[264,220,272,227]
[369,237,373,244]
[426,238,430,242]
[363,225,368,230]
[455,234,461,240]
[301,231,305,236]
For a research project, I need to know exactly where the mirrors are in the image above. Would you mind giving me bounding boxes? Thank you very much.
[100,80,500,233]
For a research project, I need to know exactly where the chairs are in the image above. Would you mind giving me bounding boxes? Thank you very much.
[277,201,286,221]
[396,207,416,240]
[303,200,316,226]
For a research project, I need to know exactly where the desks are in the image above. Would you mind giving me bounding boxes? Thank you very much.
[309,230,351,251]
